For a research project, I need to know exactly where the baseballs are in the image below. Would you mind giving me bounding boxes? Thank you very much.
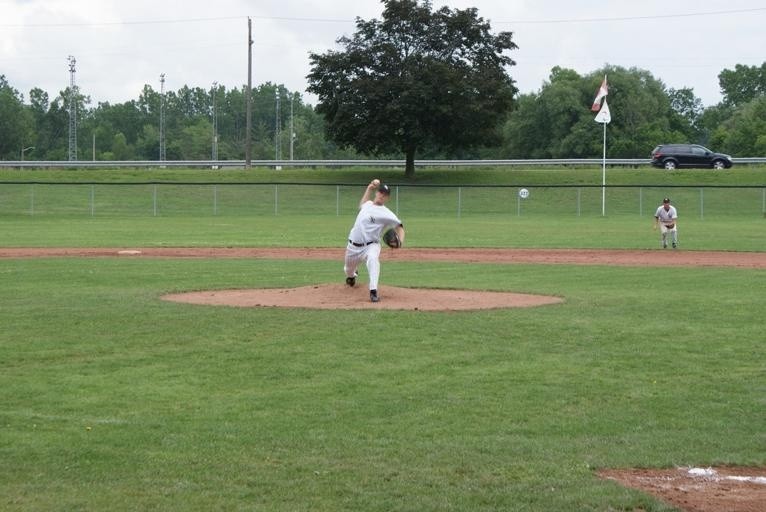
[373,179,379,186]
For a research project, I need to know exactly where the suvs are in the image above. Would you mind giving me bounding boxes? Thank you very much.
[648,143,734,171]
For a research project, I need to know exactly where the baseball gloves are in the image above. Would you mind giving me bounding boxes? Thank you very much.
[382,229,400,248]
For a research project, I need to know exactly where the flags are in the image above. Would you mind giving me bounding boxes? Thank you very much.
[590,79,612,125]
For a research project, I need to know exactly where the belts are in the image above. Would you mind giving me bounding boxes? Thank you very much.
[349,239,373,247]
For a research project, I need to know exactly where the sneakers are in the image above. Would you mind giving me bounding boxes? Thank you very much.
[346,270,358,287]
[369,289,379,301]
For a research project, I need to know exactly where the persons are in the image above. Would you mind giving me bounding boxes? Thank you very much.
[344,181,404,303]
[654,198,677,248]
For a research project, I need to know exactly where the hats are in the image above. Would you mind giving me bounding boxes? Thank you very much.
[377,183,390,194]
[663,198,670,203]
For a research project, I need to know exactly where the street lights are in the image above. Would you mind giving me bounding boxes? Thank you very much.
[210,79,220,169]
[65,54,78,161]
[288,94,295,161]
[272,86,281,169]
[157,71,168,168]
